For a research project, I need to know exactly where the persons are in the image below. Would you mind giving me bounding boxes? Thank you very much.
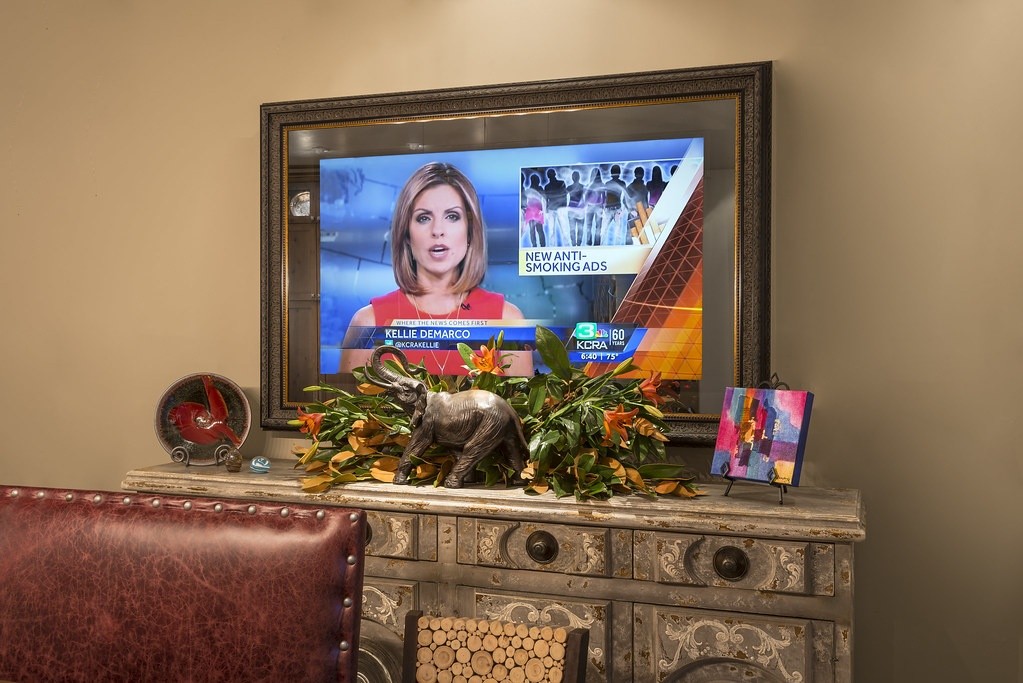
[338,161,534,380]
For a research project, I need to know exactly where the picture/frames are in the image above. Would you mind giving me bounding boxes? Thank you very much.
[259,61,773,449]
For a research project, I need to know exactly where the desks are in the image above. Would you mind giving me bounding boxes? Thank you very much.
[116,457,867,683]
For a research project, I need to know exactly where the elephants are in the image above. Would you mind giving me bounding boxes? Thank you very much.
[364,344,532,489]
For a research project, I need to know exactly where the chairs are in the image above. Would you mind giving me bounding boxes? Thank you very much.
[402,611,590,683]
[1,485,369,682]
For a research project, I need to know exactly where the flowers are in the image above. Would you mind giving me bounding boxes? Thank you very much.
[286,324,711,504]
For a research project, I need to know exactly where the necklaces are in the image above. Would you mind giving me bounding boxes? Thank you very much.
[411,293,464,376]
